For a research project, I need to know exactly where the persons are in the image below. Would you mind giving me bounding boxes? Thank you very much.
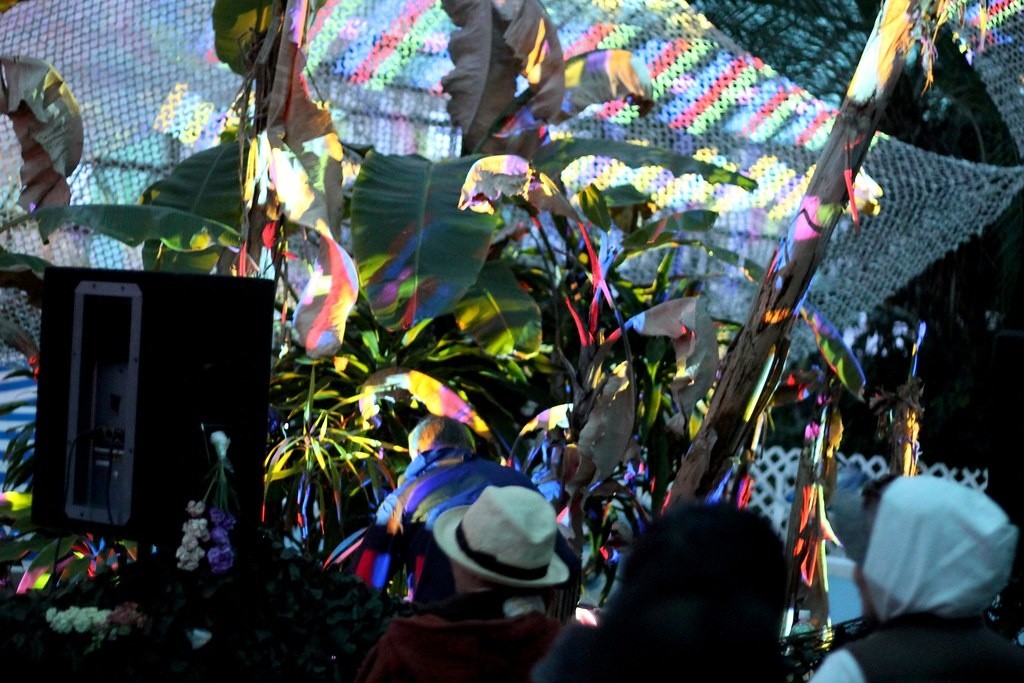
[802,472,1024,682]
[528,500,798,682]
[353,484,571,682]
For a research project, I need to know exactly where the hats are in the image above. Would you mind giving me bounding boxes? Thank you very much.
[434,484,570,585]
